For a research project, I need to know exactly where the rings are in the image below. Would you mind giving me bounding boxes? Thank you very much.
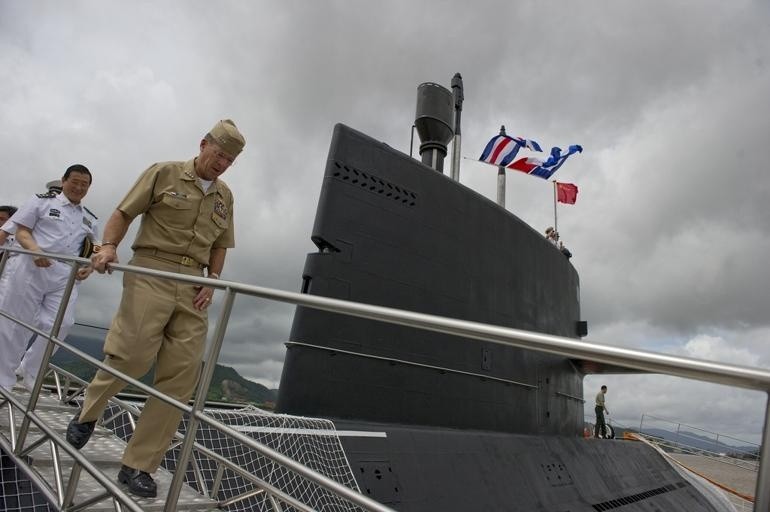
[205,297,210,302]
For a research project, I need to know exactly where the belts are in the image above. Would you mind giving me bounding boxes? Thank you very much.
[135,247,205,269]
[46,253,72,268]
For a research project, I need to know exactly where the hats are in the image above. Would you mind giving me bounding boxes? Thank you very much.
[46,180,63,194]
[80,237,94,258]
[210,118,246,158]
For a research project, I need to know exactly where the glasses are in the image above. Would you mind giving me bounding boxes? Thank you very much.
[215,151,234,166]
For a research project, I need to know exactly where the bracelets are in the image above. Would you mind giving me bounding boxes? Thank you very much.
[208,271,219,279]
[102,239,119,248]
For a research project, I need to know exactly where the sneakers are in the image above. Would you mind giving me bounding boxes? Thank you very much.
[16,369,38,393]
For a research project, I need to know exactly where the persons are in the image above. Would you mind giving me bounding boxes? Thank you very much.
[551,232,561,247]
[545,226,555,244]
[0,206,20,261]
[64,118,247,499]
[0,179,63,263]
[0,163,101,410]
[594,384,611,439]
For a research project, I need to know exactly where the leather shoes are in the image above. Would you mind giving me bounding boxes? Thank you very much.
[66,408,98,450]
[118,466,156,498]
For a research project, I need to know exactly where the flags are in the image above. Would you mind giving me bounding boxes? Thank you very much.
[477,133,583,180]
[556,183,579,205]
